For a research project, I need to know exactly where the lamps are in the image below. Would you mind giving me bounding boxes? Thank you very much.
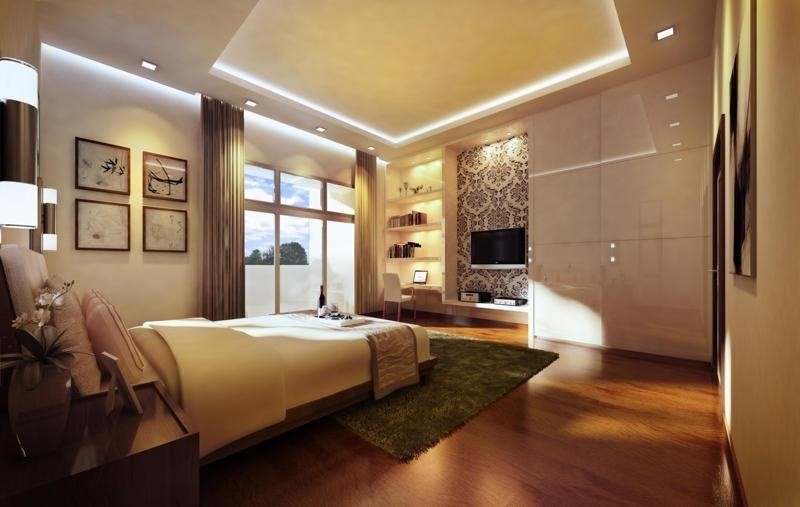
[40,187,58,251]
[0,57,38,229]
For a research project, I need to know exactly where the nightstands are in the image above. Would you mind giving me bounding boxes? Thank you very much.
[0,380,200,507]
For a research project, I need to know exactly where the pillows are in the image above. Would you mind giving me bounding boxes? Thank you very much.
[48,273,102,396]
[83,290,144,382]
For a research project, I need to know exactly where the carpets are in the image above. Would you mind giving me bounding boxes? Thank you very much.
[332,330,559,463]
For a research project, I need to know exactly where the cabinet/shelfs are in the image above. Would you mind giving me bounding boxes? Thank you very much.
[384,147,456,315]
[531,56,714,362]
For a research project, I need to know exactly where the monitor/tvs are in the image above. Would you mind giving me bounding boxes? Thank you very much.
[471,226,526,272]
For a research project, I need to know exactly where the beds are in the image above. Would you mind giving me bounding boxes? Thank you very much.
[0,243,438,469]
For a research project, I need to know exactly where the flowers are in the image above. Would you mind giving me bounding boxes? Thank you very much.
[0,277,96,391]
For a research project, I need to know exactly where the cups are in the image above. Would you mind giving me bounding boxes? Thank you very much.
[330,304,338,317]
[322,306,331,319]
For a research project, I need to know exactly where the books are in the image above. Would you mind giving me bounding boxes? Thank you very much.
[389,210,427,227]
[388,242,414,258]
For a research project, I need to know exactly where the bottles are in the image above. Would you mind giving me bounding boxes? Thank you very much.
[318,285,325,318]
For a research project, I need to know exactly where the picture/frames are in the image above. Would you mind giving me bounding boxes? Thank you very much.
[143,206,187,253]
[75,199,130,252]
[75,136,130,196]
[99,351,143,413]
[143,151,187,202]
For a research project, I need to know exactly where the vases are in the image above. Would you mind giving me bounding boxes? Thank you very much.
[9,365,72,460]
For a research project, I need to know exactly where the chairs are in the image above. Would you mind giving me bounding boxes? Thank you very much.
[382,273,416,322]
